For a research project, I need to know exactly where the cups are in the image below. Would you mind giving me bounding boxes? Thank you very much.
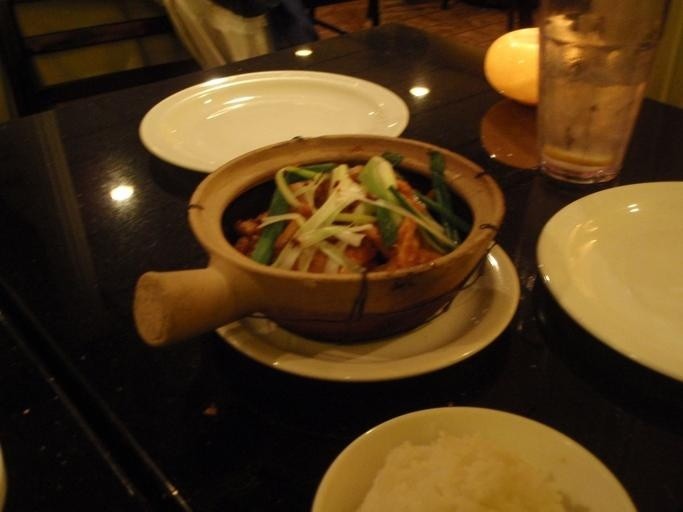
[536,1,668,181]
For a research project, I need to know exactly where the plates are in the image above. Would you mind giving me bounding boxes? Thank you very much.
[219,242,523,384]
[310,404,634,511]
[139,68,411,173]
[537,181,683,380]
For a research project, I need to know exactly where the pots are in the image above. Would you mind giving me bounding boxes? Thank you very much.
[131,134,505,344]
